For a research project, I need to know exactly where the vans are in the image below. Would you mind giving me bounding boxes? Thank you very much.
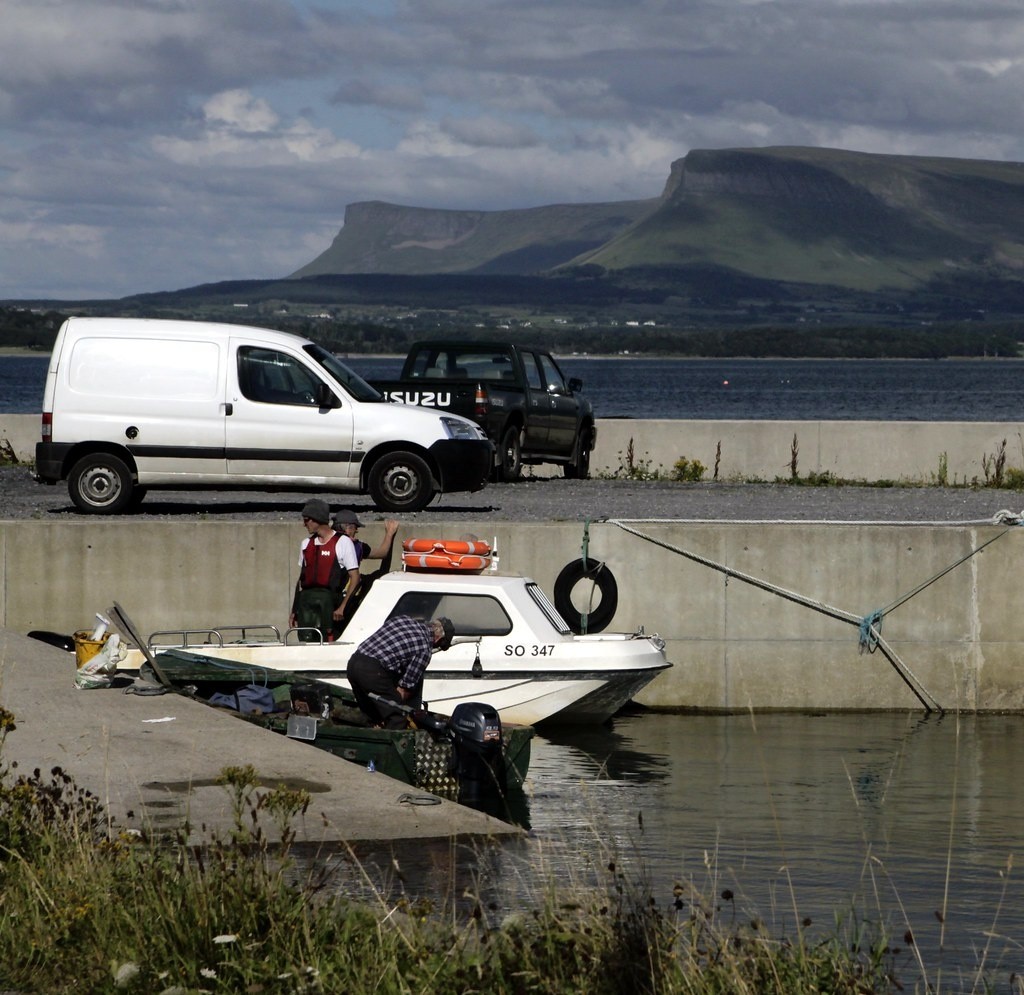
[32,316,498,514]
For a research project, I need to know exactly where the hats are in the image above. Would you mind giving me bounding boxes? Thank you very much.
[332,509,367,527]
[436,616,455,651]
[302,498,330,525]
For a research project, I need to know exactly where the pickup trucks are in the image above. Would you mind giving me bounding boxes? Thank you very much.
[363,339,599,480]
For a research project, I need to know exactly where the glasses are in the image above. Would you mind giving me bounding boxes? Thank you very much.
[302,518,311,522]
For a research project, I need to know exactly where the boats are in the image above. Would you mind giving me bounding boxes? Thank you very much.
[140,648,534,815]
[113,539,679,740]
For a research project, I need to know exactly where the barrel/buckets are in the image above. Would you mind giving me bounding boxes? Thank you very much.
[73,628,111,669]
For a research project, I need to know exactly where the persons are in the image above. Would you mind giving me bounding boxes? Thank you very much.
[347,615,455,730]
[289,499,361,642]
[331,510,399,625]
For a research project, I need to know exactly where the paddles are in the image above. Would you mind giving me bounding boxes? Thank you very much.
[104,600,172,688]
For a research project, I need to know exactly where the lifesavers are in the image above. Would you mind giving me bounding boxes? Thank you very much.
[552,557,617,635]
[402,555,493,570]
[402,537,492,556]
